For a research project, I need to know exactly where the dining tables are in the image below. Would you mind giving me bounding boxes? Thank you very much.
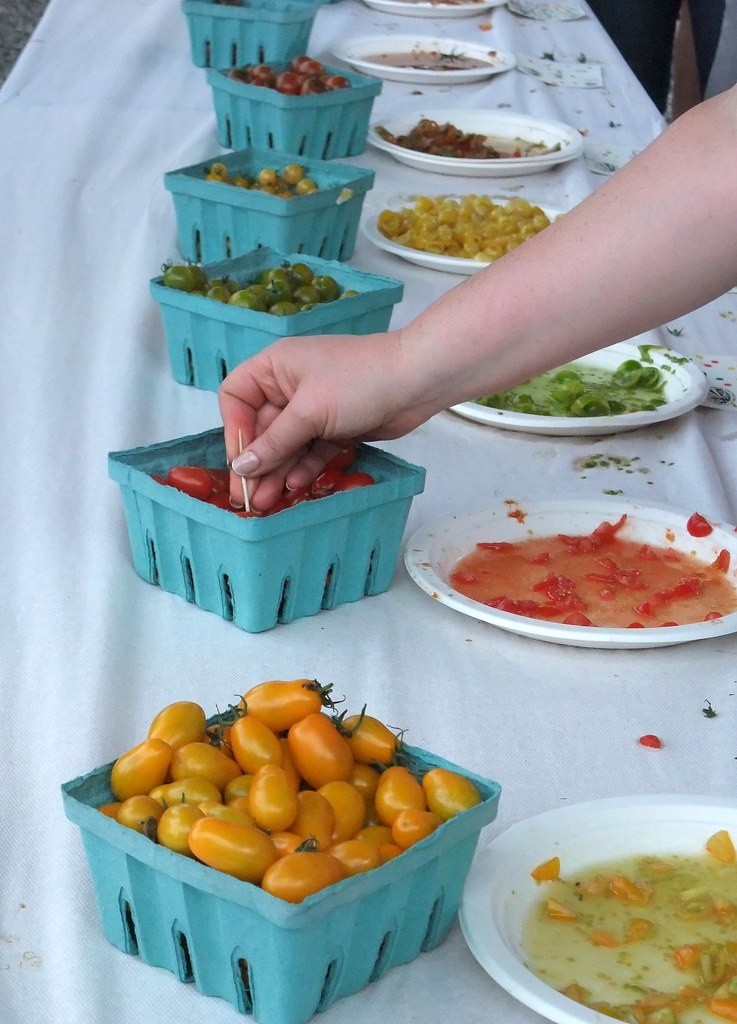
[0,0,737,1024]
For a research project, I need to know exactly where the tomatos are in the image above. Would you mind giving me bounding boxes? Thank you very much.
[229,56,351,96]
[93,678,482,905]
[201,162,319,201]
[146,441,377,520]
[157,255,360,318]
[377,194,570,262]
[470,359,664,420]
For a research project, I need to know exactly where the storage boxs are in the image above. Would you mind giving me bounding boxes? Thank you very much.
[106,0,428,637]
[59,708,500,1024]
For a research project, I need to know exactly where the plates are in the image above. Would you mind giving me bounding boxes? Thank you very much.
[403,495,737,648]
[362,198,567,276]
[448,338,709,436]
[363,0,508,18]
[332,35,516,83]
[457,794,737,1024]
[365,108,586,176]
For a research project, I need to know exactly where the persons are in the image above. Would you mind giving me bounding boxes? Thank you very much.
[215,80,737,518]
